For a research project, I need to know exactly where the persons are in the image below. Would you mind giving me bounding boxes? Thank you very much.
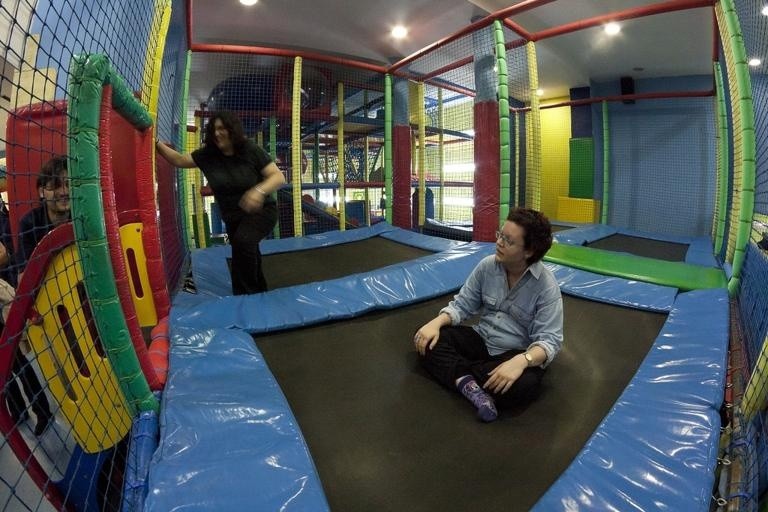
[412,205,565,423]
[1,192,54,437]
[151,109,287,296]
[16,155,74,286]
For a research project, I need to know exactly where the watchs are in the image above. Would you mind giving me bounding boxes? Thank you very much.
[523,352,533,368]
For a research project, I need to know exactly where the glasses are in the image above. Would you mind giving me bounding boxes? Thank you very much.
[495,230,526,249]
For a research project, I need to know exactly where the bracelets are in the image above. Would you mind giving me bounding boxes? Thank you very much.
[155,139,160,144]
[254,185,267,195]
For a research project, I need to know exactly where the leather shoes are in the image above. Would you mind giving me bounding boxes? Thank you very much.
[10,411,55,436]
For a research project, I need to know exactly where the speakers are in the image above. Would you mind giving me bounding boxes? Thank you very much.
[621,76,636,104]
